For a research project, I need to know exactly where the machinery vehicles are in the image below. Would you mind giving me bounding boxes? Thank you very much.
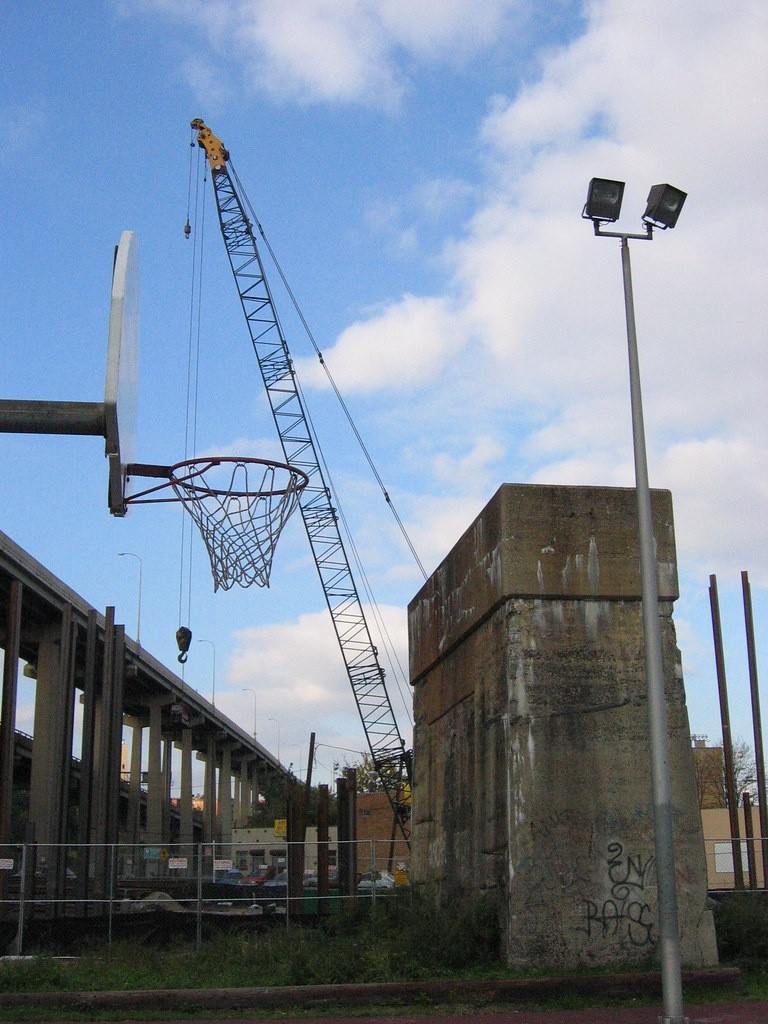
[186,117,416,874]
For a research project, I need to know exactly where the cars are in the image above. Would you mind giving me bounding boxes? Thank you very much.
[214,871,400,892]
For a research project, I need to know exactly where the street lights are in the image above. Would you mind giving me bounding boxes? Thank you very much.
[579,176,697,1024]
